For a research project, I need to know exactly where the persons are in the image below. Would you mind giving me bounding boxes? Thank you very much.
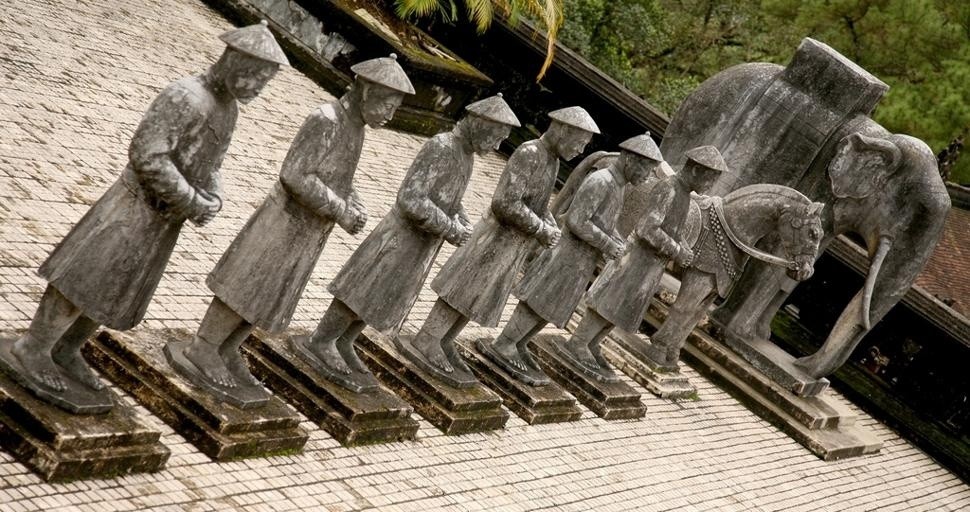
[490,131,663,372]
[409,106,602,375]
[182,54,417,390]
[302,91,521,378]
[564,143,729,371]
[10,19,289,392]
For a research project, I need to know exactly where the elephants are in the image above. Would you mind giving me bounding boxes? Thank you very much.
[658,61,952,380]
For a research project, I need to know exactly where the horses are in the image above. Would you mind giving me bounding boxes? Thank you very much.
[525,150,826,365]
